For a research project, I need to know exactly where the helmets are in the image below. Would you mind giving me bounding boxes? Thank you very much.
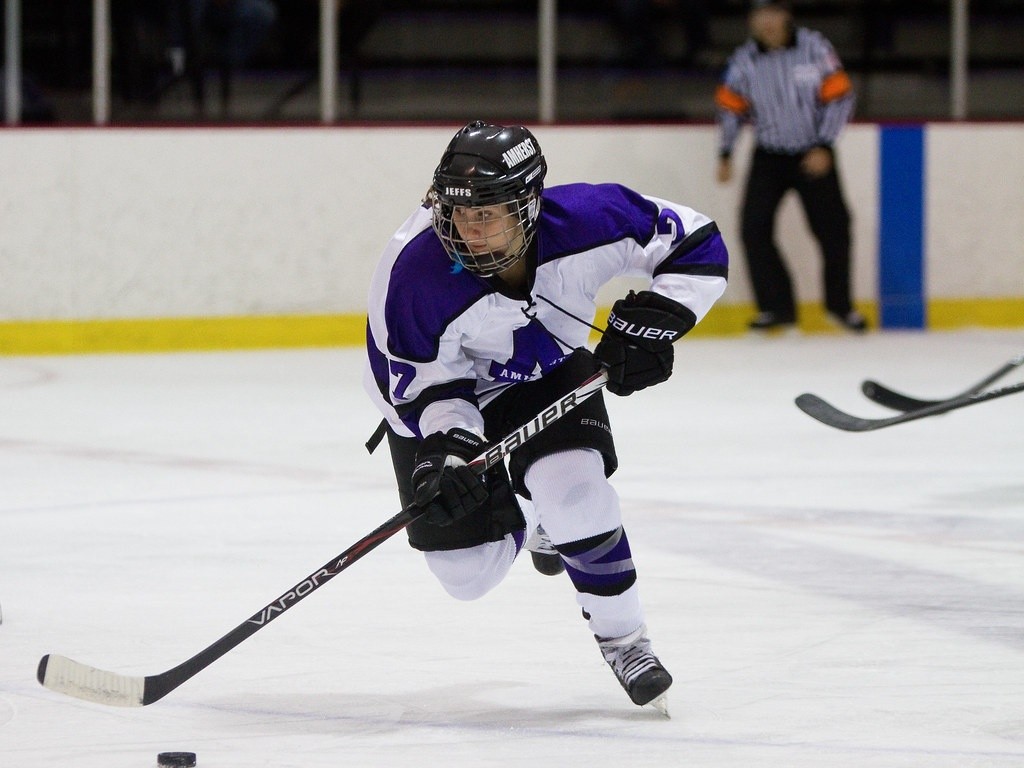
[421,121,546,278]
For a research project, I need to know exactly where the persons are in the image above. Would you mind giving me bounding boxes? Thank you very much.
[718,0,870,332]
[366,121,729,719]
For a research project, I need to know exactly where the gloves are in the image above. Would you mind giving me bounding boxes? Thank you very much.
[412,428,491,527]
[594,287,697,396]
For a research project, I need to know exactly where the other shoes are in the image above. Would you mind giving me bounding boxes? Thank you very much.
[749,312,797,334]
[828,307,867,335]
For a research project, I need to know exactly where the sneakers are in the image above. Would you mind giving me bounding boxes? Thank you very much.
[530,522,565,575]
[583,608,673,720]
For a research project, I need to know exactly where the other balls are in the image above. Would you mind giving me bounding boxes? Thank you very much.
[156,751,198,768]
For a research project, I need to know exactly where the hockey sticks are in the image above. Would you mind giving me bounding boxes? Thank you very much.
[794,381,1024,433]
[860,352,1024,416]
[35,367,611,709]
